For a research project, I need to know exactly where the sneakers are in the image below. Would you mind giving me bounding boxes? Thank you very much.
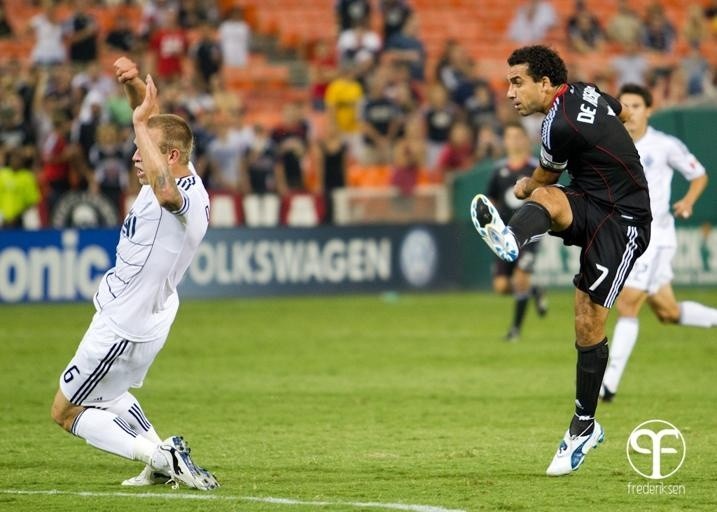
[502,326,523,342]
[536,292,550,316]
[468,192,521,265]
[120,435,188,485]
[547,416,604,477]
[150,434,221,492]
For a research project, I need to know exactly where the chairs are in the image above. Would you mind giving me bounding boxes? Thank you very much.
[0,0,717,230]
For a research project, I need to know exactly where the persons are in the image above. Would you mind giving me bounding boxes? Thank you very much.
[479,121,550,343]
[49,56,231,493]
[466,43,649,479]
[1,0,717,228]
[596,79,716,410]
